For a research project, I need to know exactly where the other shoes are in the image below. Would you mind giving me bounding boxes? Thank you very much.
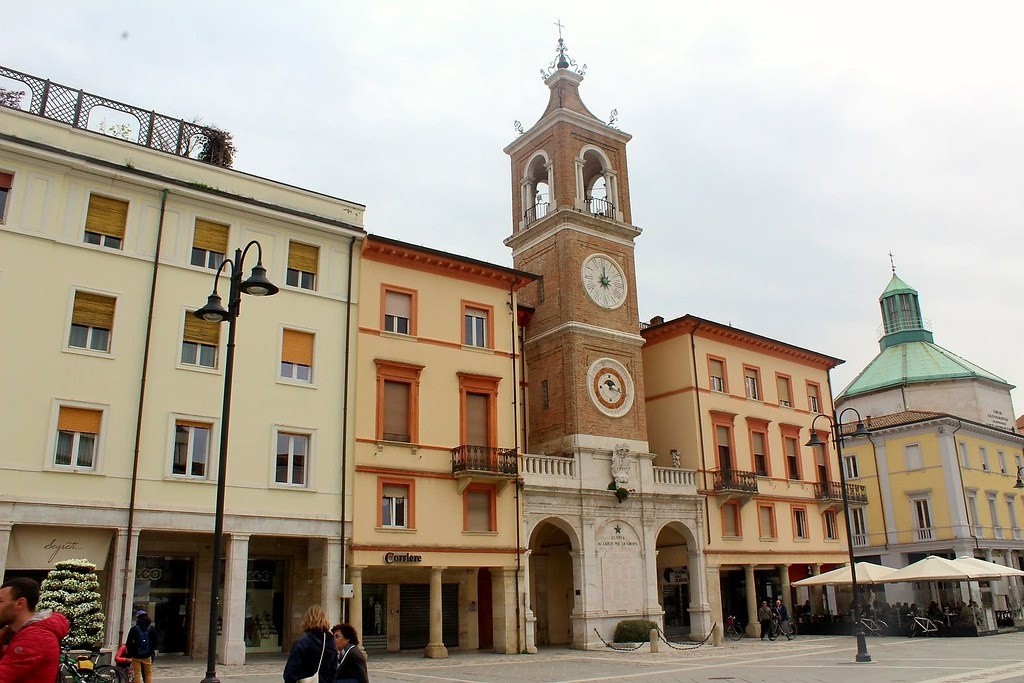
[761,638,765,641]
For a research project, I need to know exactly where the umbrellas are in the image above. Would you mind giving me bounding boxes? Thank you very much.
[874,555,1001,606]
[953,556,1024,625]
[792,562,899,616]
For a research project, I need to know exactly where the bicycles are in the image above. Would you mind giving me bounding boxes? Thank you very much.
[767,615,798,641]
[723,615,744,641]
[58,641,128,683]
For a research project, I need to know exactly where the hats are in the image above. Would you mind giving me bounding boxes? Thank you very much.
[137,610,145,617]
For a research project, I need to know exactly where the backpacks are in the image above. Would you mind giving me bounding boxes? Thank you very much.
[135,625,152,654]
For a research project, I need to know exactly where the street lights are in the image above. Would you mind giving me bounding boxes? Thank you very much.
[192,240,281,683]
[804,408,872,661]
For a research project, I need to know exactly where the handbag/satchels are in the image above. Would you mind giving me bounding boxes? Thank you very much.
[297,672,319,683]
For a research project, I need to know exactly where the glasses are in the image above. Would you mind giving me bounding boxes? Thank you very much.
[334,634,344,639]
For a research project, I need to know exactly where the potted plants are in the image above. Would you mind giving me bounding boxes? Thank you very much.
[36,559,112,676]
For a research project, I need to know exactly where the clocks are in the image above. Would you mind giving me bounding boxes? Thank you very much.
[581,252,628,310]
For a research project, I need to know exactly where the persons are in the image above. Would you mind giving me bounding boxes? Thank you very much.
[759,601,774,641]
[772,600,790,637]
[126,610,157,683]
[0,576,71,683]
[283,605,337,683]
[882,600,981,637]
[803,600,811,623]
[115,643,132,669]
[331,624,369,683]
